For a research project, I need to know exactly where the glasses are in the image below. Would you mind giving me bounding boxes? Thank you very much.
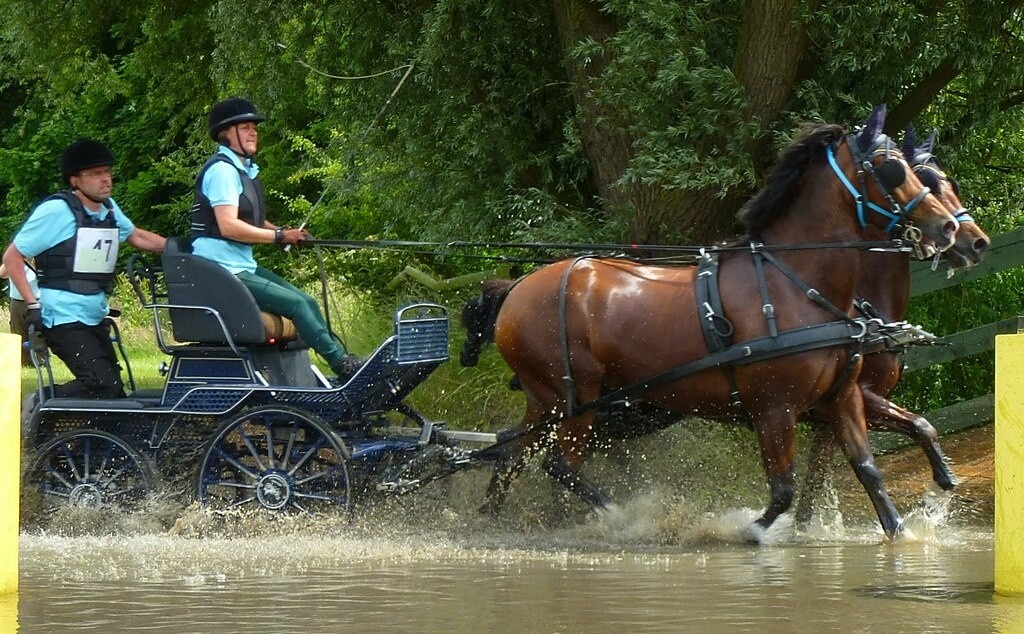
[79,169,112,178]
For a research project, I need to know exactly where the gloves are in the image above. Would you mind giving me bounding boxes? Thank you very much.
[176,237,194,254]
[23,303,42,337]
[275,226,309,244]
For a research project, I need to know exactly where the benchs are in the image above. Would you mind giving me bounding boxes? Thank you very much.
[161,235,310,353]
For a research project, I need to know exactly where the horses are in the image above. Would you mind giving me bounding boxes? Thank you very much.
[479,100,991,546]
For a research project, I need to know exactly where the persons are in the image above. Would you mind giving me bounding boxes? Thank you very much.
[3,139,168,399]
[0,256,51,368]
[192,99,369,381]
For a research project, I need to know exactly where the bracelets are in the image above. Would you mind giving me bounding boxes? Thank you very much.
[275,229,282,243]
[27,303,41,309]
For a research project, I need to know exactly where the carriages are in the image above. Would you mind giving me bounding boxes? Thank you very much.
[1,103,992,548]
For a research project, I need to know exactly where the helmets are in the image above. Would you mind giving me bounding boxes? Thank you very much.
[61,138,115,186]
[208,97,265,142]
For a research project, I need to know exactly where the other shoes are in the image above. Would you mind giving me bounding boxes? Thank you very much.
[333,353,369,386]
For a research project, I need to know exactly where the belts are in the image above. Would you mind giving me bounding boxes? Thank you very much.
[13,298,40,302]
